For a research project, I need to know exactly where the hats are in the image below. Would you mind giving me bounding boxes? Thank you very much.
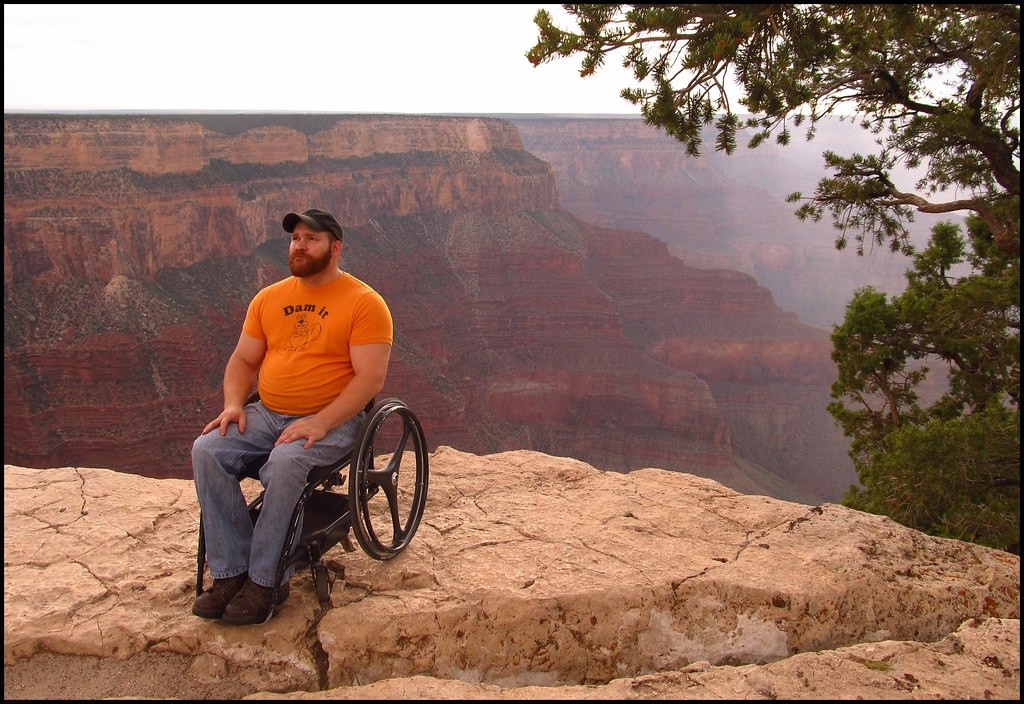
[282,208,343,243]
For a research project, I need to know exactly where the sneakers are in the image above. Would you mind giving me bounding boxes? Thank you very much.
[192,571,249,620]
[222,577,289,623]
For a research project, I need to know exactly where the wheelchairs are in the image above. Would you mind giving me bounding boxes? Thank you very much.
[195,385,430,603]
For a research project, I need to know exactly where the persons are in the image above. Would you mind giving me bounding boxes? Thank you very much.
[191,208,393,625]
